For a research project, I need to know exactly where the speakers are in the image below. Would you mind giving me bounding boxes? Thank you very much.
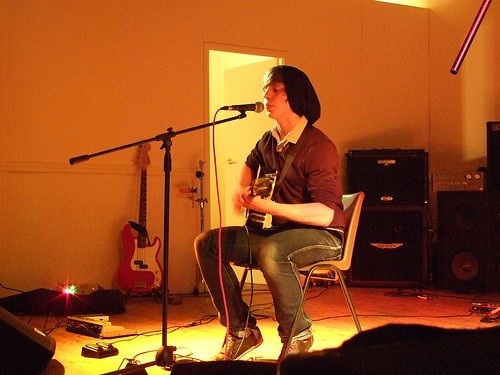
[0,306,57,375]
[435,120,500,293]
[345,205,429,288]
[347,150,428,203]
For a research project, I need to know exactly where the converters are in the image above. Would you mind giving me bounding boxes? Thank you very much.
[66,316,105,339]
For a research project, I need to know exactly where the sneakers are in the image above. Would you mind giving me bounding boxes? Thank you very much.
[278,329,314,362]
[214,326,264,362]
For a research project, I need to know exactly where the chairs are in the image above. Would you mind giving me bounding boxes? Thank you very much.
[222,192,365,368]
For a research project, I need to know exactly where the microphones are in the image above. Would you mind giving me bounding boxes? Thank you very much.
[220,102,264,113]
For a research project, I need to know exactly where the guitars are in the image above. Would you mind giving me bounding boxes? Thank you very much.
[117,143,163,294]
[245,164,278,231]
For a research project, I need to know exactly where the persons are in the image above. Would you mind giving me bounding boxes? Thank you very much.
[193,66,347,360]
